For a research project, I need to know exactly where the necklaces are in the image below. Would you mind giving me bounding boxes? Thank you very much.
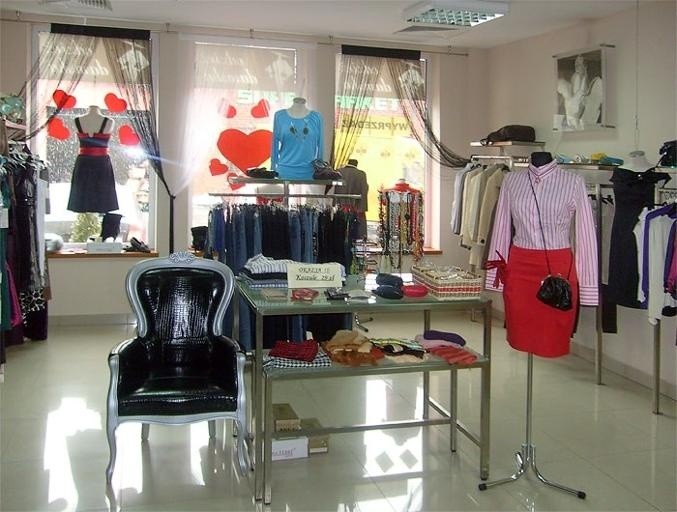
[377,191,425,269]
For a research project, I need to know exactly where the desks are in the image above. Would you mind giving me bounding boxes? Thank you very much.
[229,273,494,505]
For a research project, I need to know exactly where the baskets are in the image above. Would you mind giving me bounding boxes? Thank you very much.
[411,265,484,302]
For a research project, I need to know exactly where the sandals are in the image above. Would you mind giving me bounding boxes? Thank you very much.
[130,236,150,251]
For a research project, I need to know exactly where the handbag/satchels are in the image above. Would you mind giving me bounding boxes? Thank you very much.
[247,166,279,178]
[480,124,535,145]
[536,275,572,311]
[311,158,342,180]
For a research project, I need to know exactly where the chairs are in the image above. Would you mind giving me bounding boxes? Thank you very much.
[102,253,254,511]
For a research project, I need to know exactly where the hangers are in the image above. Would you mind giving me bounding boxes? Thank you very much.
[588,193,613,205]
[1,138,52,181]
[639,190,677,212]
[469,155,510,172]
[212,193,359,216]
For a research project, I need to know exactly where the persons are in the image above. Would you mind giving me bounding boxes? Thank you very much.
[557,54,604,130]
[335,159,367,248]
[270,98,323,181]
[66,106,119,212]
[485,152,600,357]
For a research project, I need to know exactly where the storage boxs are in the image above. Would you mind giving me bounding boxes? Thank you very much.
[271,403,331,461]
